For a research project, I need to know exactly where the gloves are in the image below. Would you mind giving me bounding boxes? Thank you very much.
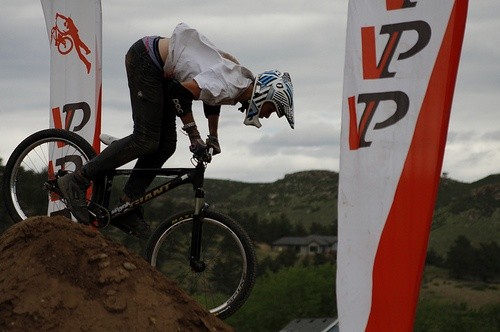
[206,133,220,154]
[188,131,206,155]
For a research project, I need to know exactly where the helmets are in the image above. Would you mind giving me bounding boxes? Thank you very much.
[244,70,294,128]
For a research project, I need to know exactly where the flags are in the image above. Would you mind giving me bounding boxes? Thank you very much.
[40,0,103,220]
[337,1,469,331]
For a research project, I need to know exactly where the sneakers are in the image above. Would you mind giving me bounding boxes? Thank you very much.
[57,170,90,225]
[111,202,144,233]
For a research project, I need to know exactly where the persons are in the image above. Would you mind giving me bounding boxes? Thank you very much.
[56,23,296,238]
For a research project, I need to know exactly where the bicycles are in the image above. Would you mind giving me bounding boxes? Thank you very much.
[49,15,73,56]
[3,126,256,320]
